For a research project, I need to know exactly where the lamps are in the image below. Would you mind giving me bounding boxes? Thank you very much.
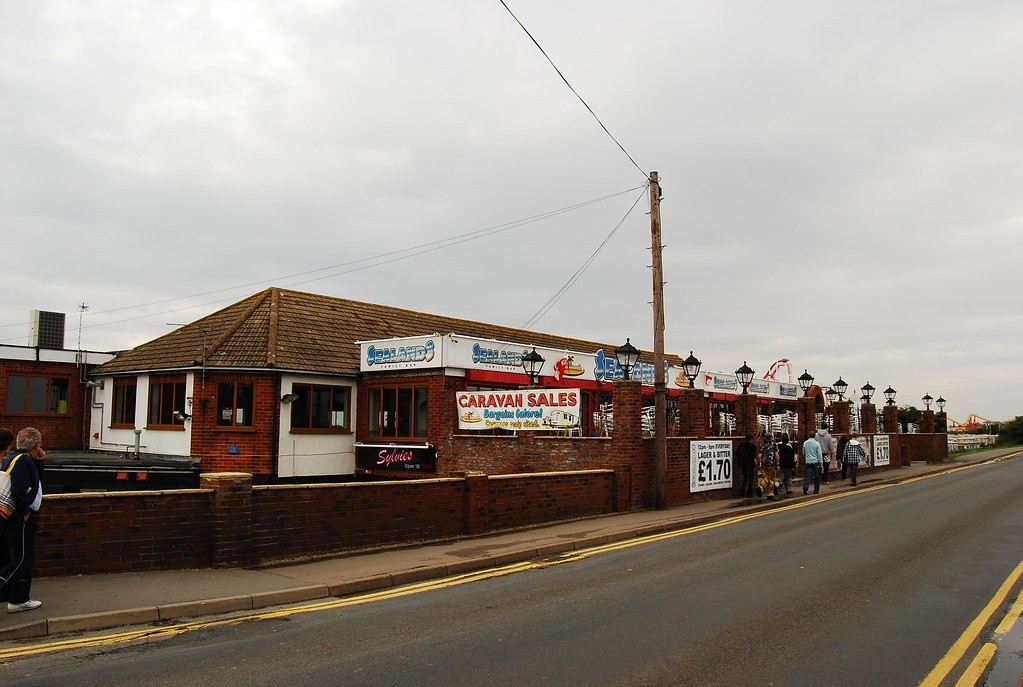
[847,398,855,416]
[735,361,755,394]
[521,346,546,385]
[936,396,947,412]
[682,350,702,387]
[833,377,848,401]
[824,386,836,405]
[922,393,933,411]
[883,385,897,406]
[173,410,191,422]
[281,394,300,403]
[614,338,641,380]
[860,381,875,403]
[797,369,814,397]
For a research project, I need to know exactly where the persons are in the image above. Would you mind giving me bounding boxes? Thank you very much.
[757,432,797,502]
[802,431,823,495]
[735,435,759,498]
[0,427,46,614]
[835,432,866,486]
[814,423,835,485]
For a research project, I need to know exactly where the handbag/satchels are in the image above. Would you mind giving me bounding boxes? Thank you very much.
[857,456,869,469]
[0,452,32,520]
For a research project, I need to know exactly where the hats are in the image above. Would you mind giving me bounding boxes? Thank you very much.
[763,432,774,442]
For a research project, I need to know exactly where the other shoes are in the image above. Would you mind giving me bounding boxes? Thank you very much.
[739,477,863,498]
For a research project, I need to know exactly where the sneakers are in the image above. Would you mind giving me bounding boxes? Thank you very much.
[7,599,41,613]
